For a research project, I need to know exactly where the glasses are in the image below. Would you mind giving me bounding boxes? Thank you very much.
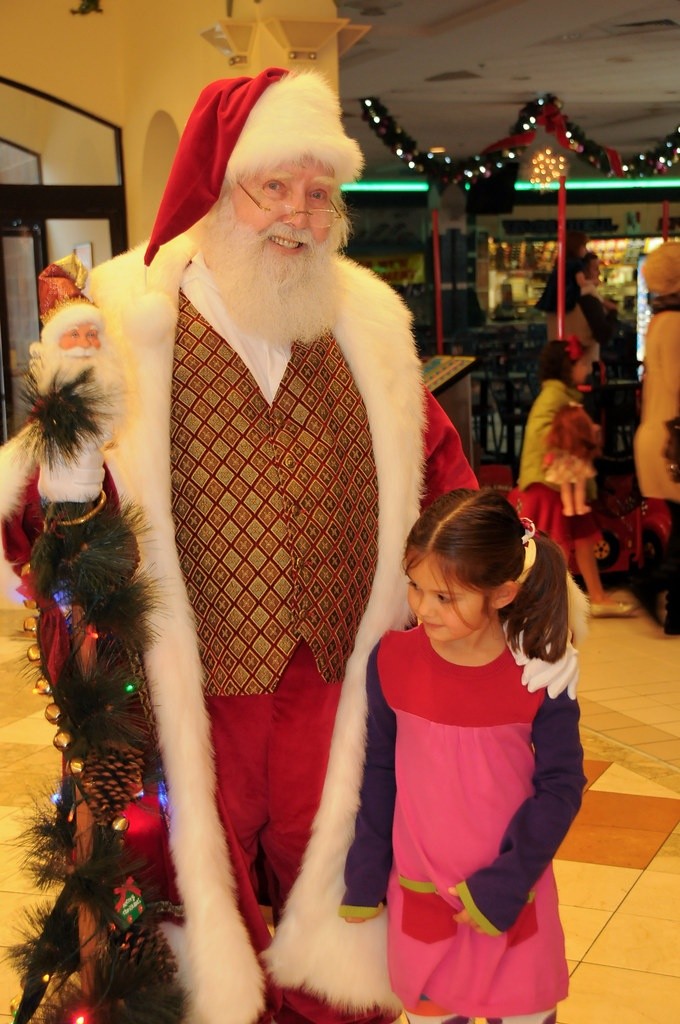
[236,182,342,227]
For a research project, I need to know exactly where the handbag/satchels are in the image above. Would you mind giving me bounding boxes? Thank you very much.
[664,417,680,481]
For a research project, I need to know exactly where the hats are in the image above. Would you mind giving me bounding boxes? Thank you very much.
[643,243,680,296]
[144,67,365,345]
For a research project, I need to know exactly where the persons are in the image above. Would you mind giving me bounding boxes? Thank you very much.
[516,340,636,617]
[29,305,121,435]
[0,66,588,1024]
[634,239,680,637]
[535,228,627,498]
[339,487,586,1024]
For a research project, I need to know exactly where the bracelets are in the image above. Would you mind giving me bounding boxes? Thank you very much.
[55,490,105,525]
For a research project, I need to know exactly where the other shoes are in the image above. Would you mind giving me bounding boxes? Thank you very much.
[665,614,680,634]
[591,602,638,617]
[631,570,668,625]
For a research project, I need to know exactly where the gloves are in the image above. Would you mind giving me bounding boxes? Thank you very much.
[503,614,581,700]
[37,421,109,503]
